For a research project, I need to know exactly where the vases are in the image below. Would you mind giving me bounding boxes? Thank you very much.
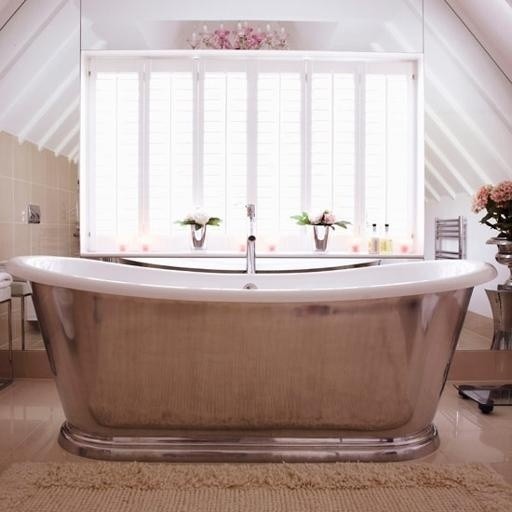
[191,225,206,248]
[314,225,329,251]
[485,238,512,290]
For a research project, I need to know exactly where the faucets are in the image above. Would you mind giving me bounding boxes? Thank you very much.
[245,235,256,274]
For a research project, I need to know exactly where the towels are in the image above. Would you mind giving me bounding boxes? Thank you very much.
[0,260,13,288]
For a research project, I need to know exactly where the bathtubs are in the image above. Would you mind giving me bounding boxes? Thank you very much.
[5,254,499,461]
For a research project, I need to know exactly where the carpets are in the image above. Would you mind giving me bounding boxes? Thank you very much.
[0,459,512,512]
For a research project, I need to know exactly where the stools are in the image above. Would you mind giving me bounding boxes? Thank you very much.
[11,282,32,351]
[0,281,13,392]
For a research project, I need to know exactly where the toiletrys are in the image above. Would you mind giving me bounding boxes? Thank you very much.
[367,224,379,256]
[379,223,393,255]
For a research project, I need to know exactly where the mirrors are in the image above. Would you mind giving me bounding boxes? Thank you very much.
[0,1,81,351]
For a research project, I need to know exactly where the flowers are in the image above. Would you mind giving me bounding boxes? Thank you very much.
[469,180,512,237]
[173,211,223,230]
[290,209,354,232]
[186,21,290,49]
